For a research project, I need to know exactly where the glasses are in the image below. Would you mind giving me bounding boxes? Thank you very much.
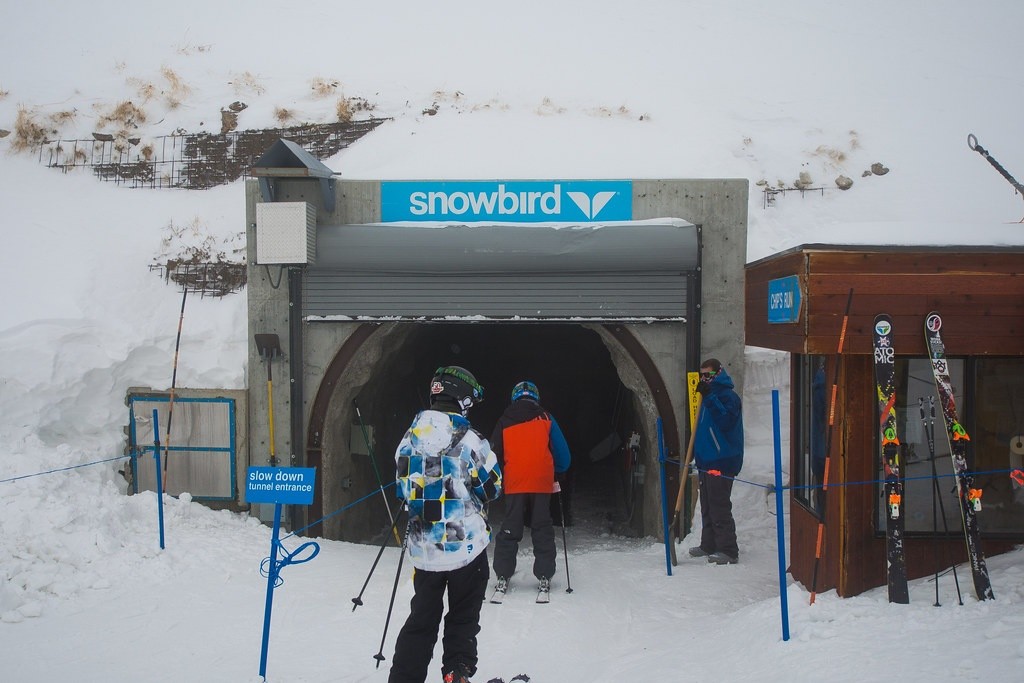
[699,370,716,379]
[472,385,488,405]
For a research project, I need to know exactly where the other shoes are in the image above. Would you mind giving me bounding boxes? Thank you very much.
[497,576,507,592]
[539,580,550,592]
[689,546,710,557]
[708,551,739,565]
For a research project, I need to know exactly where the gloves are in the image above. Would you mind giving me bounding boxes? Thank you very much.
[695,380,712,397]
[554,472,560,483]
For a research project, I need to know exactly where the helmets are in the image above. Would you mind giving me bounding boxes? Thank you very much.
[429,365,479,418]
[510,381,540,402]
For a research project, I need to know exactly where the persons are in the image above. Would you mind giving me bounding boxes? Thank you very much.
[491,381,570,589]
[388,365,503,683]
[689,358,744,564]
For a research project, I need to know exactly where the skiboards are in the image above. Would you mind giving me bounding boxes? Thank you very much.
[870,308,996,605]
[489,576,550,604]
[487,673,529,683]
